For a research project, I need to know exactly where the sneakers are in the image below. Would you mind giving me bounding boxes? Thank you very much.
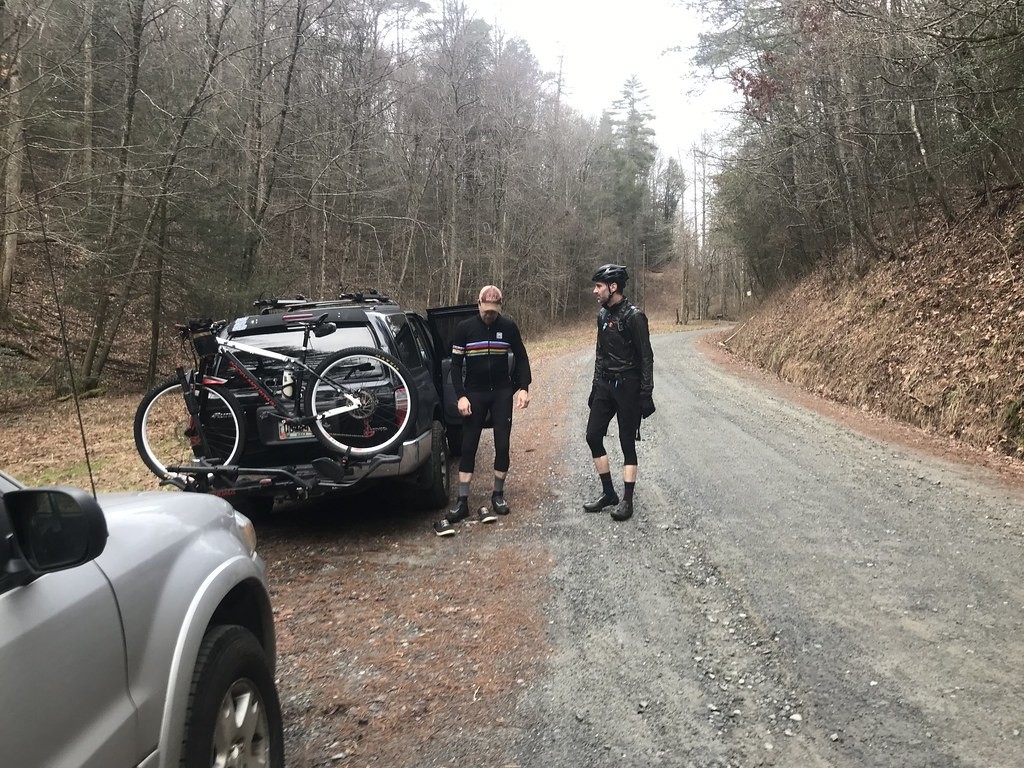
[478,506,497,523]
[446,501,470,524]
[433,519,456,537]
[492,495,510,515]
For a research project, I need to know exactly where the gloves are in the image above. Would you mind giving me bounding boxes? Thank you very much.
[641,399,655,419]
[588,385,597,409]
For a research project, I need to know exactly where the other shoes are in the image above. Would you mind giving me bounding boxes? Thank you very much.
[609,500,633,521]
[583,493,619,512]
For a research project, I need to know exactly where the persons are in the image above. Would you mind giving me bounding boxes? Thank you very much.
[582,265,655,520]
[445,285,531,523]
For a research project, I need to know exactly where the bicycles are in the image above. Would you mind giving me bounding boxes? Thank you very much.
[131,310,416,489]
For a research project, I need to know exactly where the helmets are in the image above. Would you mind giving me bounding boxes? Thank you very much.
[592,264,629,282]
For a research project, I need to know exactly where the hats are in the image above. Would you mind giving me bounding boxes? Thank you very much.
[478,285,502,313]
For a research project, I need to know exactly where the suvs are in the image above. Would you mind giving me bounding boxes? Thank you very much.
[0,465,285,768]
[185,293,490,518]
